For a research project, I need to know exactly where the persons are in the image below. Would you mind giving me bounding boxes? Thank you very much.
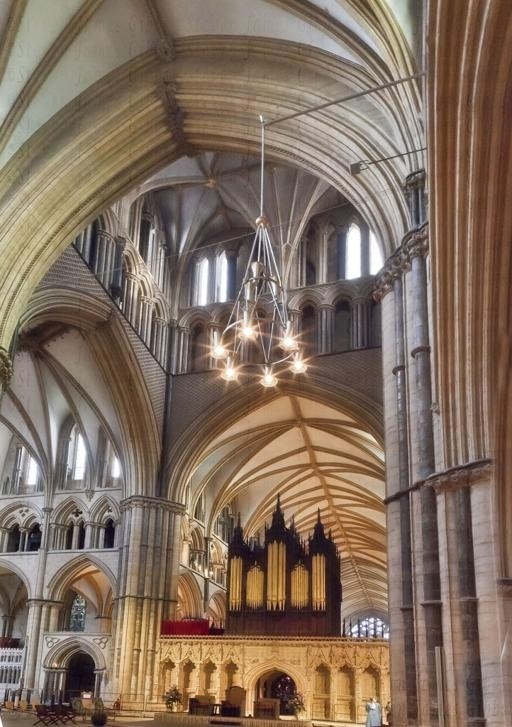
[365,696,382,727]
[386,696,392,726]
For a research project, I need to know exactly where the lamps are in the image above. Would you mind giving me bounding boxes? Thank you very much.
[211,124,309,385]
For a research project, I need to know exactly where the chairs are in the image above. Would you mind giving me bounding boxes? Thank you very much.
[33,704,79,726]
